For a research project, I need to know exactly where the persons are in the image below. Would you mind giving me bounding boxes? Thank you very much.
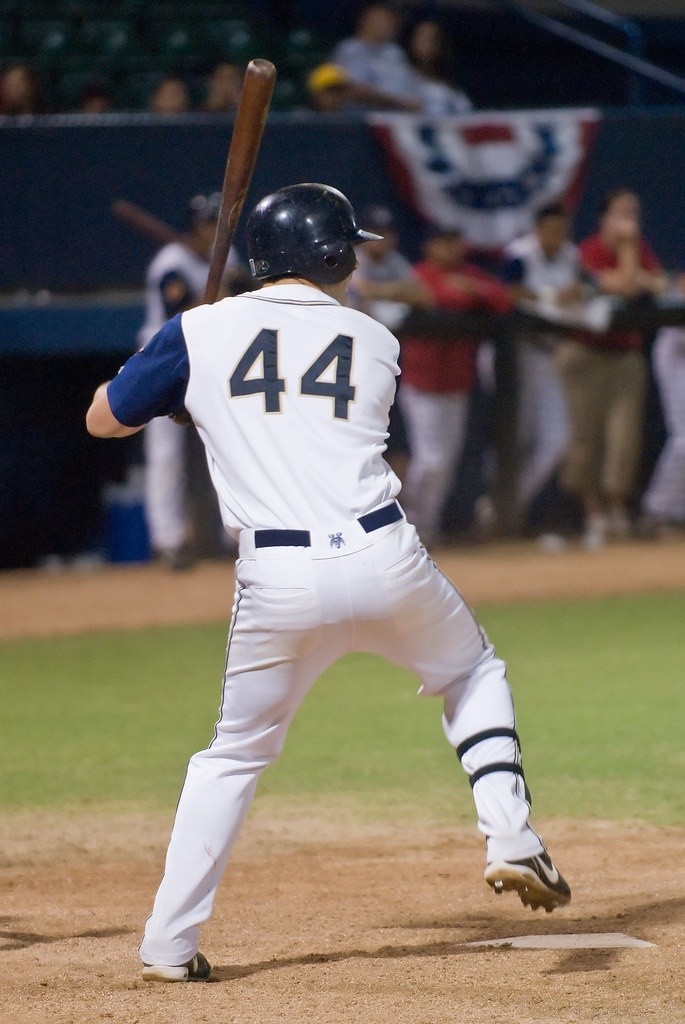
[85,183,572,981]
[1,0,685,566]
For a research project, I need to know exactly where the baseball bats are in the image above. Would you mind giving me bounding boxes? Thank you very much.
[198,55,280,309]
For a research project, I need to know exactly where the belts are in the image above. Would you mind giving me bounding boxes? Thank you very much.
[255,502,403,548]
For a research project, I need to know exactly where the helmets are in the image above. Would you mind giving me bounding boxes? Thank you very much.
[243,182,384,285]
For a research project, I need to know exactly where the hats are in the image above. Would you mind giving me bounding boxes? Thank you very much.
[308,62,350,91]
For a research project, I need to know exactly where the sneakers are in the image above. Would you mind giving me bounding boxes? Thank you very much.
[142,951,212,982]
[484,852,571,912]
